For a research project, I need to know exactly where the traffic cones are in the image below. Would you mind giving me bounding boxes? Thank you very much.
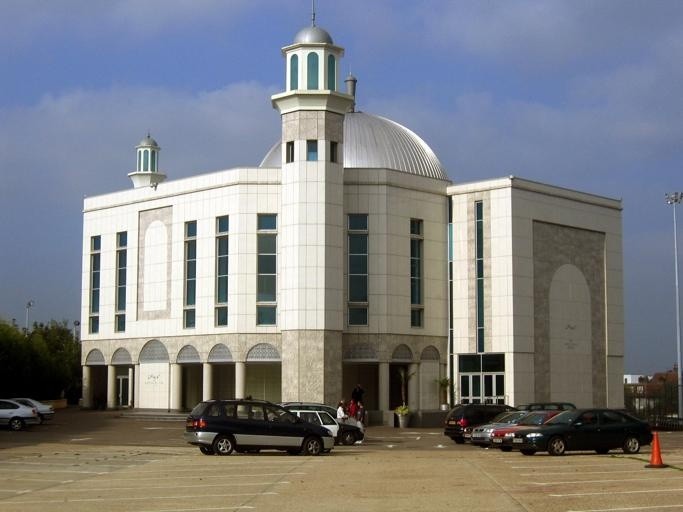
[645,432,669,468]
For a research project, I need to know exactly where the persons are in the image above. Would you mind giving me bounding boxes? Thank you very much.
[337,400,364,446]
[352,383,364,404]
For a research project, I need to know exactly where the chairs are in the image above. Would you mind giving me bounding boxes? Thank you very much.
[229,408,262,420]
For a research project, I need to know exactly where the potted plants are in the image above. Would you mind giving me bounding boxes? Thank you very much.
[394,405,413,429]
[434,377,452,411]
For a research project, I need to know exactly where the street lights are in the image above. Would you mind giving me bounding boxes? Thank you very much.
[25,300,34,337]
[72,320,80,354]
[662,190,682,427]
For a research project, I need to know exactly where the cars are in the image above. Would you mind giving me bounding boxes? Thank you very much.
[184,399,364,456]
[0,397,55,431]
[443,401,683,455]
